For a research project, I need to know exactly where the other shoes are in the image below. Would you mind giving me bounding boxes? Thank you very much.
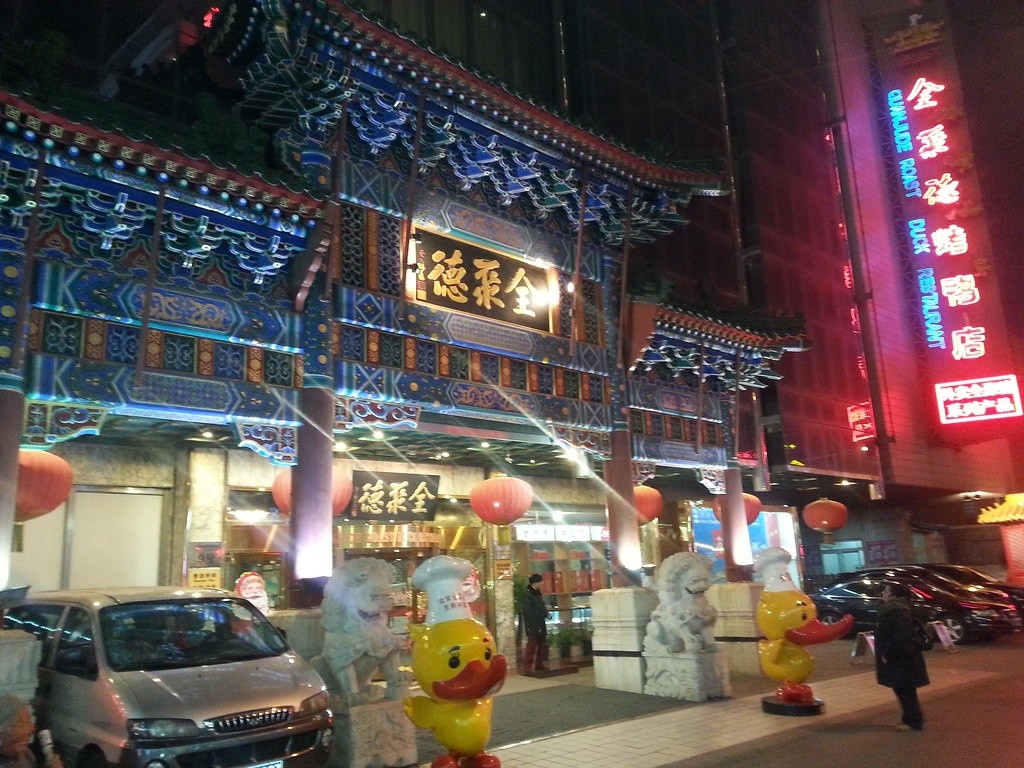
[527,671,537,676]
[538,665,549,670]
[896,723,913,731]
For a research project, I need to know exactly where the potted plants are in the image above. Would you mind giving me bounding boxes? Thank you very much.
[555,624,577,659]
[544,633,555,660]
[576,628,594,655]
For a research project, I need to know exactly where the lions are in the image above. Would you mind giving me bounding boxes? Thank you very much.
[640,553,721,654]
[308,558,405,707]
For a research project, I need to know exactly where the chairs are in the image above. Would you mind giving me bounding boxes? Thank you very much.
[167,610,205,650]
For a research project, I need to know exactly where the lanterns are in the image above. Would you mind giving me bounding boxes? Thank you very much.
[11,448,74,551]
[633,484,664,543]
[803,498,849,543]
[470,477,534,545]
[272,465,354,517]
[712,492,762,526]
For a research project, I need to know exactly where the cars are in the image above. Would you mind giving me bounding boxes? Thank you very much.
[0,584,336,768]
[808,562,1024,646]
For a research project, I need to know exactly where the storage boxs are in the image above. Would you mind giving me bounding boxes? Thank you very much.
[552,571,563,593]
[530,551,550,560]
[542,571,554,593]
[576,570,591,592]
[568,550,586,560]
[563,570,577,592]
[590,569,601,591]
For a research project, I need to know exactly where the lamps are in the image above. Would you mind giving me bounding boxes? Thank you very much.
[974,495,981,501]
[963,495,971,502]
[833,479,858,486]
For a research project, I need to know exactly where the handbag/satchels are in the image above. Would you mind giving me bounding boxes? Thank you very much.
[912,617,933,651]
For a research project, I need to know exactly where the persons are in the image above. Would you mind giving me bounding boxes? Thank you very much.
[874,584,932,731]
[521,574,552,675]
[198,607,275,660]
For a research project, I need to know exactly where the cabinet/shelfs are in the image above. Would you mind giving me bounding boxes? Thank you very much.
[515,542,608,650]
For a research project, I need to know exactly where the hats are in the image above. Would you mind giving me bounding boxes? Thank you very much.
[529,574,542,583]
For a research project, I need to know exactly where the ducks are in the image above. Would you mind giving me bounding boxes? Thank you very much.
[401,623,507,768]
[752,548,853,705]
